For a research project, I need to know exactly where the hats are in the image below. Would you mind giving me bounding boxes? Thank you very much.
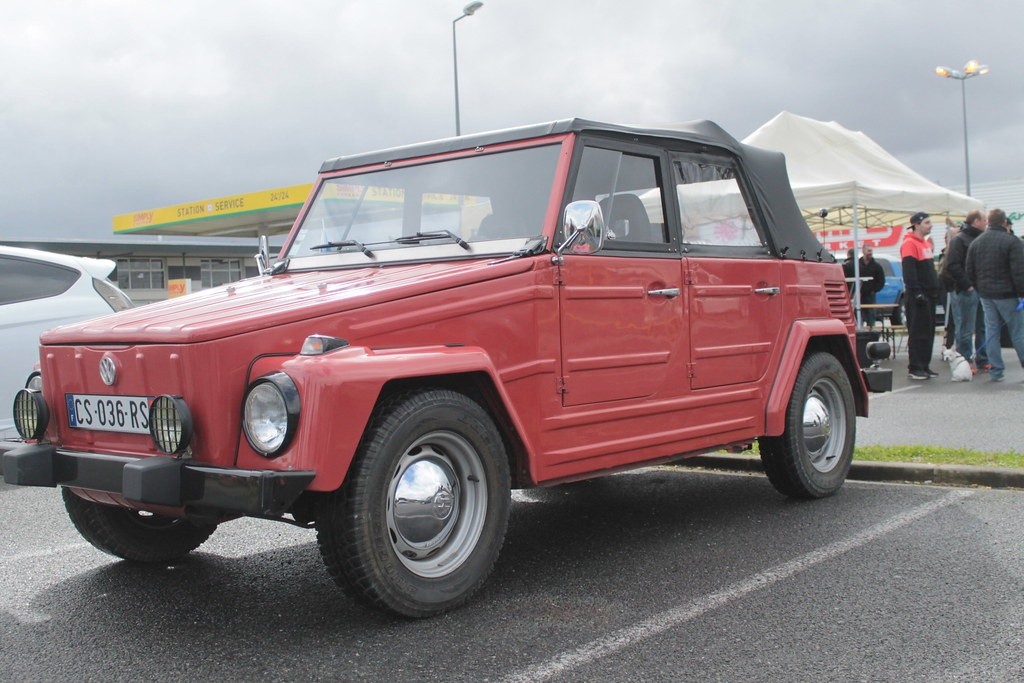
[907,212,928,229]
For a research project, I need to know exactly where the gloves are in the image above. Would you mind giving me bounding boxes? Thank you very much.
[916,295,927,306]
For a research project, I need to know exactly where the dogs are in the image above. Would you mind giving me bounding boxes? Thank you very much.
[941,343,973,383]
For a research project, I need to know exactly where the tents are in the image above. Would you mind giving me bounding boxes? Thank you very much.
[639,110,984,325]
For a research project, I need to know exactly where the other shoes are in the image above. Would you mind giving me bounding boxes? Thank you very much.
[969,364,976,375]
[993,375,1005,381]
[977,363,992,369]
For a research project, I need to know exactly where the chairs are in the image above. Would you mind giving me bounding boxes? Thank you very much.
[598,193,652,241]
[475,186,538,237]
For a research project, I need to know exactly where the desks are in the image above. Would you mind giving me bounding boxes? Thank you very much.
[859,303,900,340]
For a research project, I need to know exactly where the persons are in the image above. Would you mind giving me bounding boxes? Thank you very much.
[842,244,885,326]
[942,209,1024,381]
[900,212,939,379]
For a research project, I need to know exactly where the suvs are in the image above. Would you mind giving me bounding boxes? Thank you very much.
[833,252,906,330]
[0,244,136,443]
[0,118,871,621]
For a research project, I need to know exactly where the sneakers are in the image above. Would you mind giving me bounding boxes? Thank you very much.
[924,369,939,378]
[908,370,929,379]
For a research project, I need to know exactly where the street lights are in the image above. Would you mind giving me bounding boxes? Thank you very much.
[934,59,992,198]
[451,0,484,137]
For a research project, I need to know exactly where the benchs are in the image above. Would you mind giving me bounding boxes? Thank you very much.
[874,324,908,360]
